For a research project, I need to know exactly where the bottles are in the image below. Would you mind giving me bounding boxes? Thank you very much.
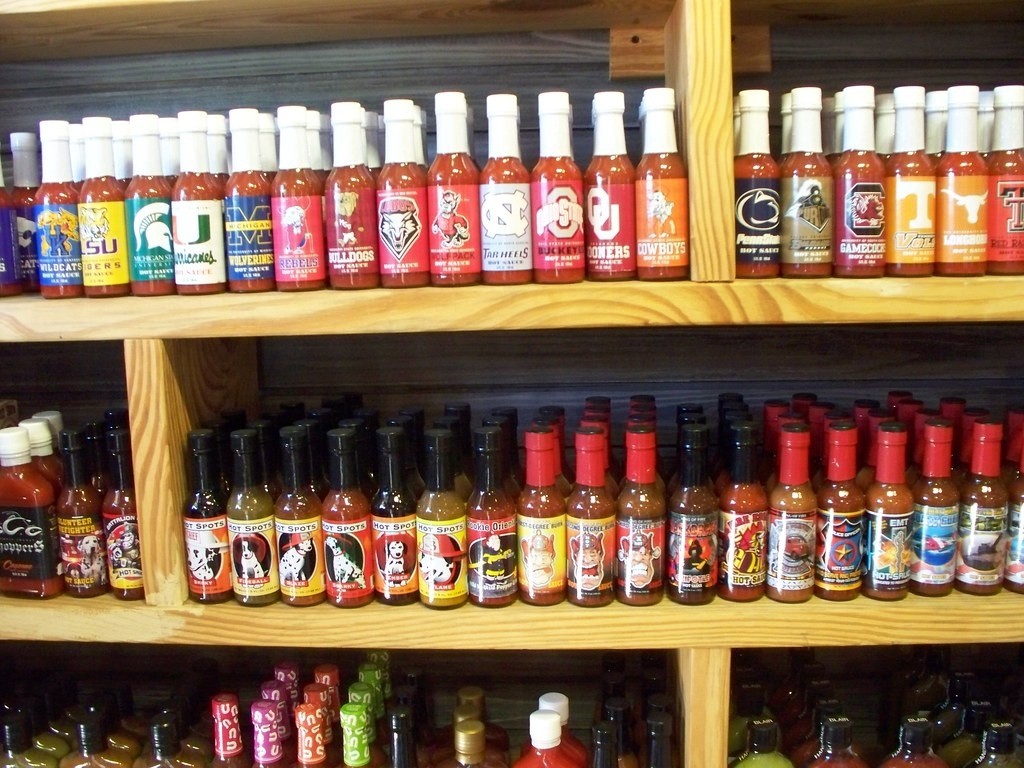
[729,643,1024,768]
[0,409,139,603]
[0,651,684,768]
[0,89,685,298]
[732,85,1024,276]
[184,394,1024,609]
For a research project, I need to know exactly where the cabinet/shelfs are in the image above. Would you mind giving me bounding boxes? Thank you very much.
[0,0,1024,768]
